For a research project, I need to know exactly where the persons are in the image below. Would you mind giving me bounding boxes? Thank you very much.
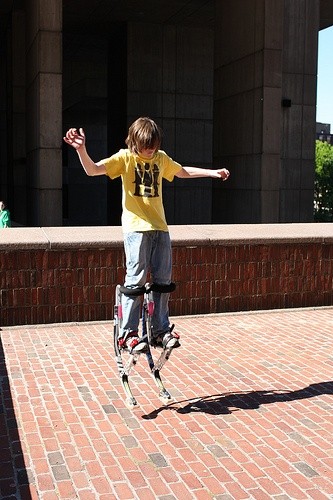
[63,117,230,353]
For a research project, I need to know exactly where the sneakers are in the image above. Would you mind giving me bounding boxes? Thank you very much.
[124,336,148,351]
[155,331,180,348]
